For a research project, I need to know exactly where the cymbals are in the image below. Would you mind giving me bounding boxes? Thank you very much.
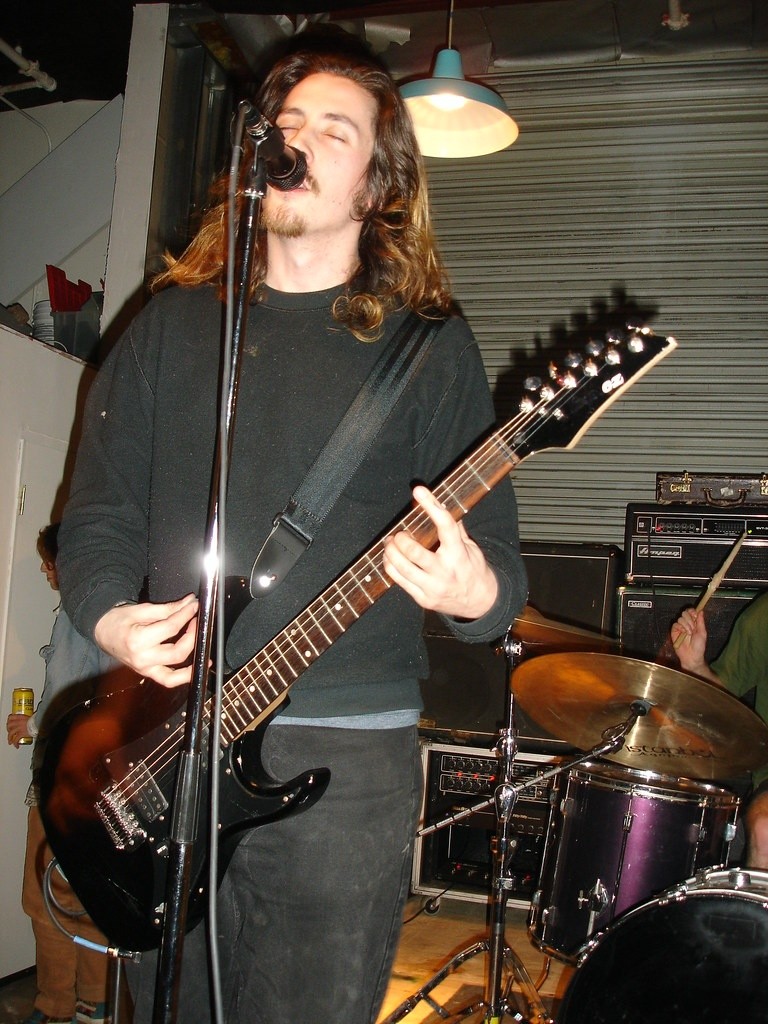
[511,651,768,781]
[510,605,623,650]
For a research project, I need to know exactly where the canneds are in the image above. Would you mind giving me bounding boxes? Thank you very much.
[11,688,34,745]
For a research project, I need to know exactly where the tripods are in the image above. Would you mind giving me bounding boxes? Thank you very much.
[380,705,648,1024]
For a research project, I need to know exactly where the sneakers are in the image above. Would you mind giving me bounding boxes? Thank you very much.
[74,999,113,1024]
[22,1008,78,1024]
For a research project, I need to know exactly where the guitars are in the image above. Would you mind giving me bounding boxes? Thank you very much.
[30,295,680,954]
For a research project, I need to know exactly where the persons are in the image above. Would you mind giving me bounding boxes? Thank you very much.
[54,48,528,1024]
[6,521,120,1024]
[670,585,768,871]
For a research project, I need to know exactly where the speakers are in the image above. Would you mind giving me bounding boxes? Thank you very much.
[413,540,756,753]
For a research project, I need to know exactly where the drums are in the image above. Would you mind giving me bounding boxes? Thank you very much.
[526,757,740,968]
[556,865,768,1024]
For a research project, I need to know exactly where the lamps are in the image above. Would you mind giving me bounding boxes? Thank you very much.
[398,0,519,159]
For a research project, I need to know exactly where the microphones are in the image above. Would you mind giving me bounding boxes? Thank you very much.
[236,99,307,192]
